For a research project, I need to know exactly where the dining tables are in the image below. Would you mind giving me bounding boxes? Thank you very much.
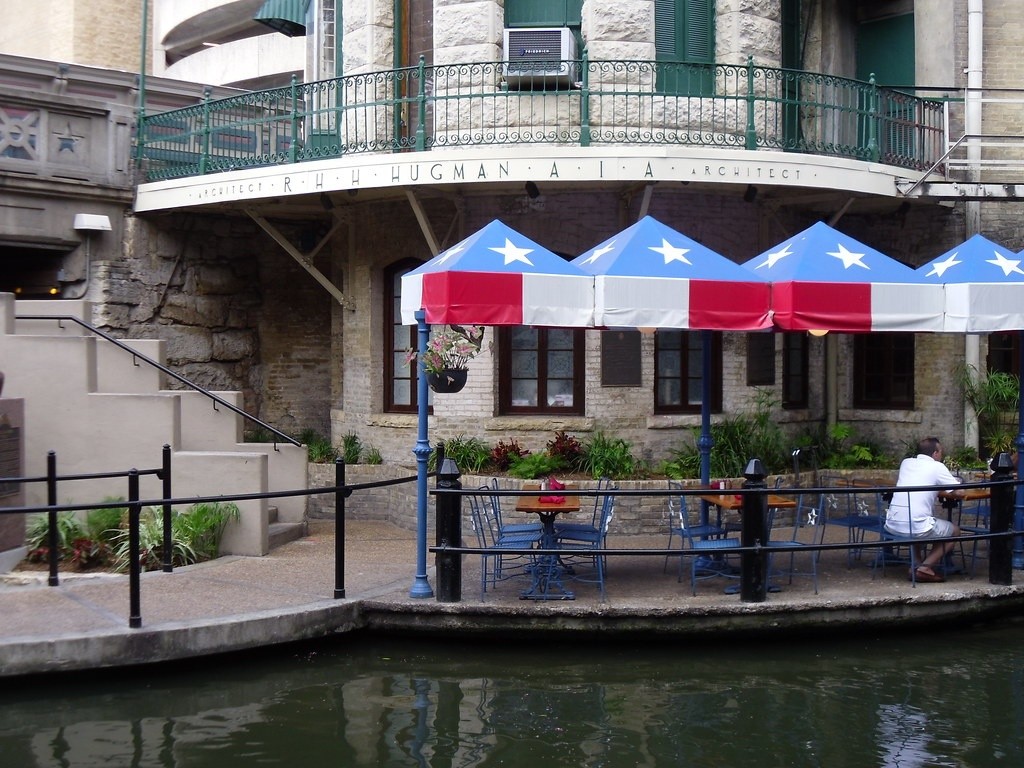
[835,476,991,583]
[515,483,581,602]
[682,484,798,596]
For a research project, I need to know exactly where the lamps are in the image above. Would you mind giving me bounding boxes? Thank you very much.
[808,329,830,337]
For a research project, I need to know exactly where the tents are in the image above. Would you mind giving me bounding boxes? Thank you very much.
[403,218,1024,597]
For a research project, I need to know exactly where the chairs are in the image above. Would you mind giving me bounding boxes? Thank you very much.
[721,476,784,543]
[661,480,727,583]
[549,484,619,584]
[871,484,950,590]
[547,476,610,568]
[954,466,993,581]
[677,495,741,597]
[848,478,889,580]
[551,487,620,598]
[765,490,829,596]
[491,478,543,569]
[466,494,545,597]
[816,475,865,572]
[478,485,545,581]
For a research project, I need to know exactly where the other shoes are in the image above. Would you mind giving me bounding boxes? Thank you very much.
[908,567,948,583]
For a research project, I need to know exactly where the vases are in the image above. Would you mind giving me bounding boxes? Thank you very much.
[421,367,469,394]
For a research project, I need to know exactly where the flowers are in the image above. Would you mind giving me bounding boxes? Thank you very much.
[402,324,497,374]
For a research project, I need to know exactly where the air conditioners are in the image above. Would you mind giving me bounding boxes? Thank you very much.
[502,26,583,91]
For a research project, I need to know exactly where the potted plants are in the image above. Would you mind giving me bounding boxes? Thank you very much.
[22,493,243,577]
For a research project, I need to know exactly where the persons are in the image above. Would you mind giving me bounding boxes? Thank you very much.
[886,438,965,582]
[876,699,937,768]
